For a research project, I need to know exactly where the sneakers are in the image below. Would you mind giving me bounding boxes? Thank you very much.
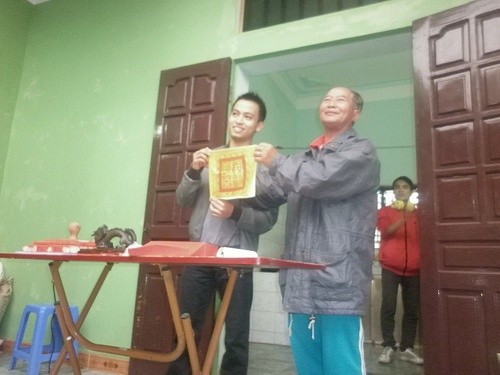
[378,346,396,364]
[398,347,423,365]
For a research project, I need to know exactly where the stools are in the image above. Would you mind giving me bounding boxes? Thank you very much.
[8,305,82,374]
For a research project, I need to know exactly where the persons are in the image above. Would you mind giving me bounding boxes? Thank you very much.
[238,88,381,375]
[375,176,424,364]
[166,92,279,375]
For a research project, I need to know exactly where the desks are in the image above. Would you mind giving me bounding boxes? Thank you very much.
[0,251,326,375]
